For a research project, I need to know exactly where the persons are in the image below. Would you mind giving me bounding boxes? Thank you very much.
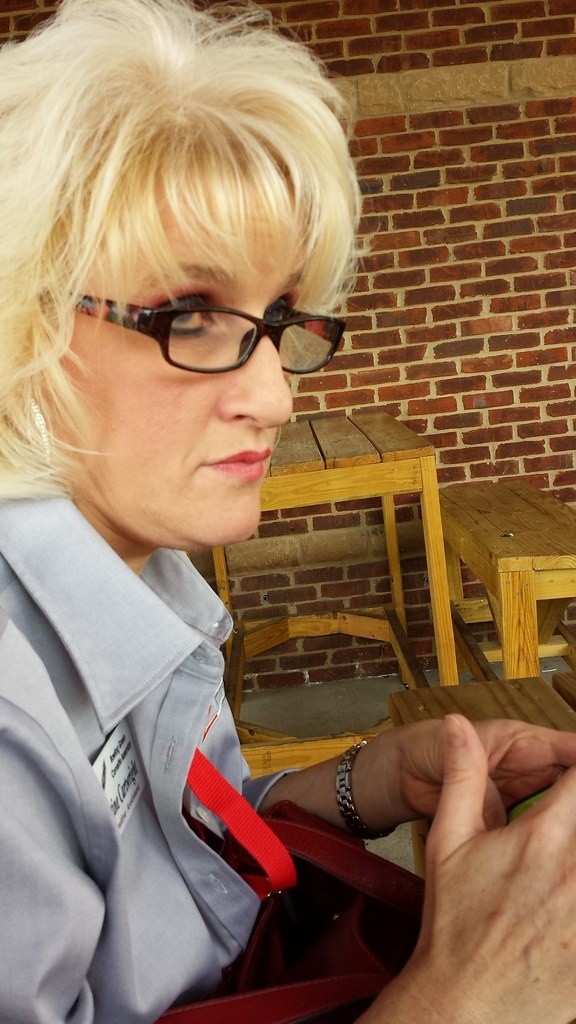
[0,0,575,1024]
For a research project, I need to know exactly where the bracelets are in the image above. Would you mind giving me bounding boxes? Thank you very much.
[336,740,397,839]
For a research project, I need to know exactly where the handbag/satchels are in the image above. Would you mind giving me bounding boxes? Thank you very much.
[153,801,426,1024]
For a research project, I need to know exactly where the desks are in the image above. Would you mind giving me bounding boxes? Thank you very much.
[209,410,459,747]
[388,670,576,874]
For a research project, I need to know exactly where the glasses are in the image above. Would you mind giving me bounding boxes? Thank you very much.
[42,289,346,375]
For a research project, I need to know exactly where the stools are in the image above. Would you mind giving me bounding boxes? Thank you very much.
[437,481,576,680]
